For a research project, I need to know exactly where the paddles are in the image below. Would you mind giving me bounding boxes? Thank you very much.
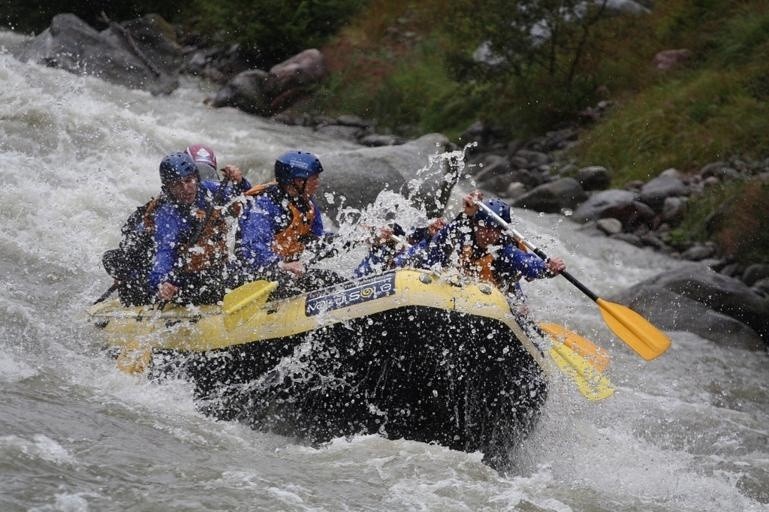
[473,200,672,361]
[221,234,341,333]
[117,175,229,373]
[520,310,617,402]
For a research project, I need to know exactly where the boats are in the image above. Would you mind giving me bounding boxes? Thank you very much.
[94,269,551,462]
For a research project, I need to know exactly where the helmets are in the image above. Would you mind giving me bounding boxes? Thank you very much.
[159,152,202,185]
[183,143,218,171]
[472,197,512,231]
[274,149,324,185]
